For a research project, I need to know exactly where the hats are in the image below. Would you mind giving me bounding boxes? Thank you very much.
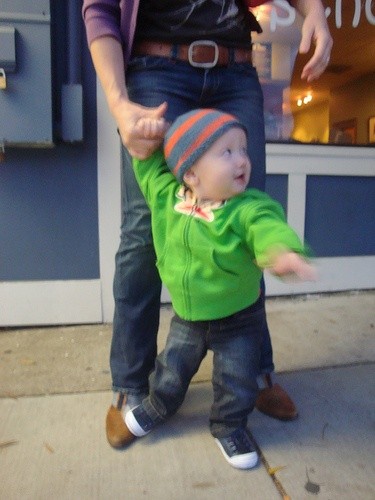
[164,109,248,182]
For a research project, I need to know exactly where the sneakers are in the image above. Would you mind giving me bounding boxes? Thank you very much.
[126,399,162,437]
[215,427,258,470]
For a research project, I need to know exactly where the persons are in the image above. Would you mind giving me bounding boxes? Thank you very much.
[82,0,335,450]
[123,110,321,470]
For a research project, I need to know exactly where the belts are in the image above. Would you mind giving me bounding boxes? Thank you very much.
[132,40,252,68]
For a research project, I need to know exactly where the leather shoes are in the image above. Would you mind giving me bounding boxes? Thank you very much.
[256,383,299,421]
[106,392,150,448]
